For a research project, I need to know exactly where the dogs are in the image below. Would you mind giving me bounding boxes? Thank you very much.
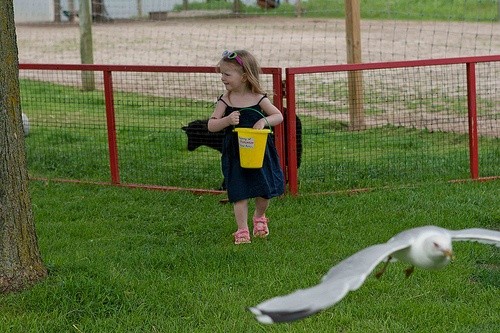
[182,104,303,192]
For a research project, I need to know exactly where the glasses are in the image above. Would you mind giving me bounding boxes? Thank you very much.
[222,50,242,64]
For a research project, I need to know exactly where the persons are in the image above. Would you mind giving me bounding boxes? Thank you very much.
[208,50,284,244]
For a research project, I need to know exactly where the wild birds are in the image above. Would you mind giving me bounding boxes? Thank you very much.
[246,225,500,325]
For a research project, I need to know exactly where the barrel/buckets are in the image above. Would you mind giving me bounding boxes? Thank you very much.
[21,113,29,135]
[232,108,274,168]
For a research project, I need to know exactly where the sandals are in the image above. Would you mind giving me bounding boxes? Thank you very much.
[253,215,269,239]
[234,228,250,243]
